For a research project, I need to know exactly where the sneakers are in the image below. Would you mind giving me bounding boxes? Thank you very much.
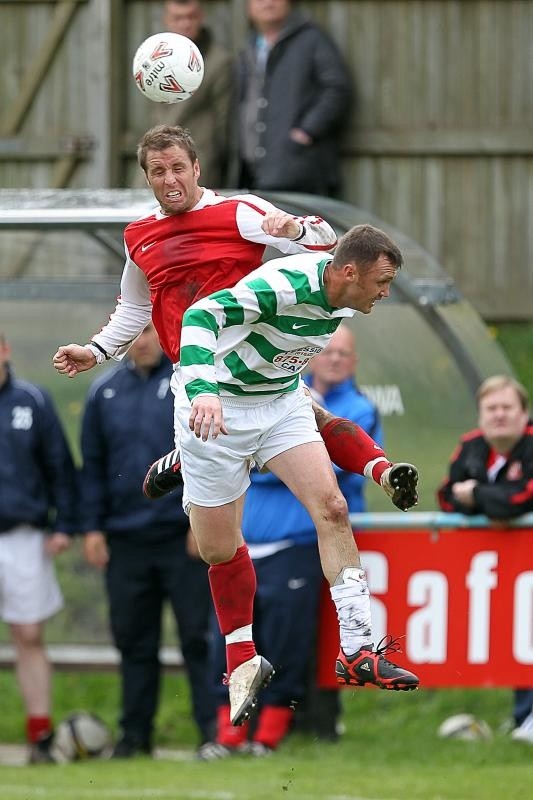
[381,462,420,512]
[142,448,181,498]
[333,634,419,691]
[221,654,275,727]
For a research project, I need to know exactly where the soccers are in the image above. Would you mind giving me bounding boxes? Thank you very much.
[50,708,118,765]
[131,32,207,104]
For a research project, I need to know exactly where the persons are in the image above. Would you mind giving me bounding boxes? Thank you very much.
[79,318,234,762]
[282,318,388,740]
[436,373,533,743]
[223,0,358,193]
[191,466,326,765]
[133,0,234,184]
[171,221,423,726]
[1,332,77,772]
[50,125,424,518]
